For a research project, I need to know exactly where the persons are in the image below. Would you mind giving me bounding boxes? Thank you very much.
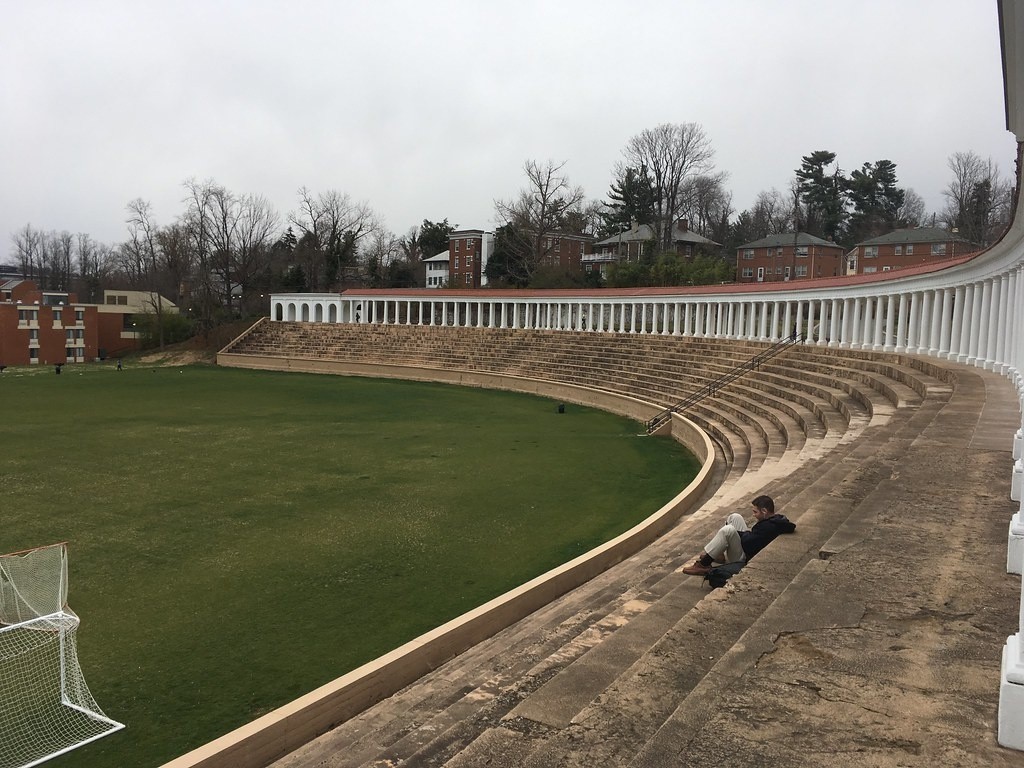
[117,359,122,371]
[356,313,360,323]
[682,495,796,575]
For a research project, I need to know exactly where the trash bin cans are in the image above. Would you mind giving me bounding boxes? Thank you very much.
[559,403,564,413]
[56,366,60,374]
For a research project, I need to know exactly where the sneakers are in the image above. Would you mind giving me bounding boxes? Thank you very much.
[683,560,712,576]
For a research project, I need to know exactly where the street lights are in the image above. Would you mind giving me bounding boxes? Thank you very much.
[260,294,264,317]
[238,295,242,320]
[188,307,193,323]
[133,322,138,353]
